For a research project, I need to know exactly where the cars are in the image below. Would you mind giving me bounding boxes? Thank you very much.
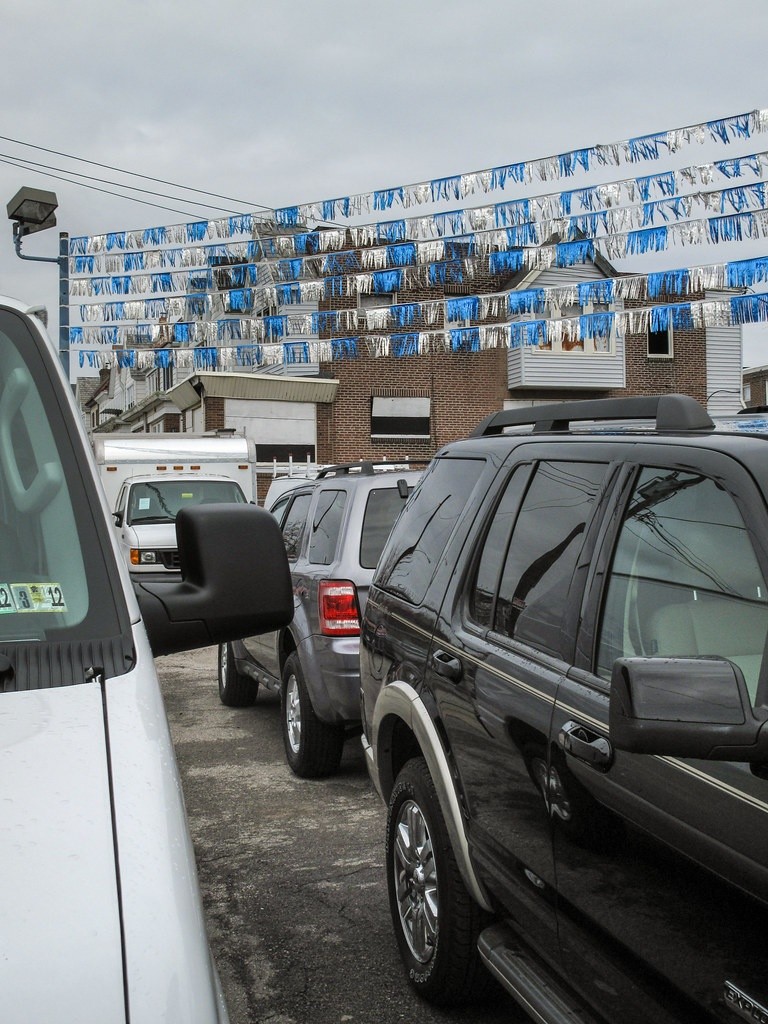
[1,294,296,1023]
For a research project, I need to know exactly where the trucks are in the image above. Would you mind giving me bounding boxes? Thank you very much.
[87,428,261,596]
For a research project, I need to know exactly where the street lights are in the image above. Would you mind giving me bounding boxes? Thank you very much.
[6,186,70,382]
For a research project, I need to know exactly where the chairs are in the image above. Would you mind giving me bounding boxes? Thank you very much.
[133,498,161,518]
[644,528,768,711]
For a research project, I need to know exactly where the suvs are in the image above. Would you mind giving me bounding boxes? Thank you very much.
[358,394,768,1024]
[217,458,434,781]
